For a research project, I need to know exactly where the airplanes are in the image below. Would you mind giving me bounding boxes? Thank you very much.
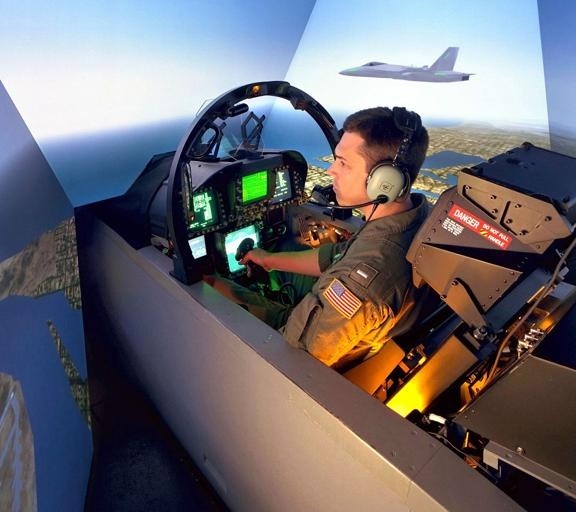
[339,41,474,87]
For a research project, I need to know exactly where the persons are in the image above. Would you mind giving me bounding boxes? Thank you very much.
[199,105,430,369]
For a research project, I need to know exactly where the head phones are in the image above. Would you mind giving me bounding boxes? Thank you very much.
[365,106,422,205]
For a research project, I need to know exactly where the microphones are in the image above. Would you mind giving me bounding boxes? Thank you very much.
[312,191,388,210]
